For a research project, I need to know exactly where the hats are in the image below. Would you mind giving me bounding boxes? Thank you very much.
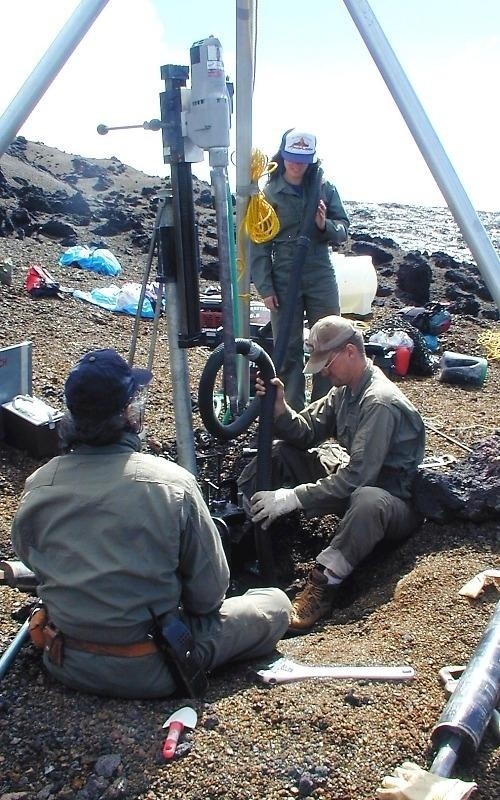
[64,349,153,415]
[281,126,320,164]
[301,314,354,376]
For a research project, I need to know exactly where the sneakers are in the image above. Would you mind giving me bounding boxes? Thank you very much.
[285,565,340,633]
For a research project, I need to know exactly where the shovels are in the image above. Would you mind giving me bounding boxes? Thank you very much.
[160,707,198,759]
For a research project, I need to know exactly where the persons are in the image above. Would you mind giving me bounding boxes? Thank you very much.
[250,129,350,415]
[236,315,425,635]
[10,349,293,700]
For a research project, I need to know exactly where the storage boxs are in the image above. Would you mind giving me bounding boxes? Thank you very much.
[2,393,65,458]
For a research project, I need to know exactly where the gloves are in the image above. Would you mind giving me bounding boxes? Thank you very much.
[376,760,478,800]
[250,487,301,532]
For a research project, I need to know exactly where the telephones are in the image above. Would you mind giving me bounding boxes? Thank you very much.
[148,608,210,698]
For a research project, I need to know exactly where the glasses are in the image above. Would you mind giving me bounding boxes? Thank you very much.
[318,351,343,379]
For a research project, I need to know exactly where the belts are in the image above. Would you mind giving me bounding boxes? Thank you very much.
[60,634,157,657]
